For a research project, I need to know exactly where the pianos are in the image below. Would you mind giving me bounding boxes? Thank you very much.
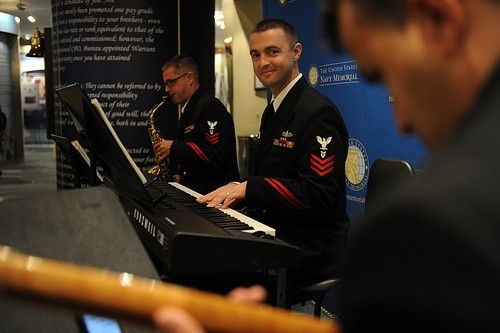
[104,175,301,316]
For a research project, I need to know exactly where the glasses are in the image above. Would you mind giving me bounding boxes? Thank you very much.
[161,72,196,88]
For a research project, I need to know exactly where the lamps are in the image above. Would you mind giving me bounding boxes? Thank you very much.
[27,25,46,58]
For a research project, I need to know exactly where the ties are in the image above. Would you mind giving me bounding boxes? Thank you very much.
[262,101,275,135]
[178,112,184,130]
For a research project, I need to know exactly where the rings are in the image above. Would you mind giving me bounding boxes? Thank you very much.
[226,192,230,196]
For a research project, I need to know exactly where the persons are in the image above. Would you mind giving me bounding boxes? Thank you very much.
[151,0,500,333]
[195,18,349,252]
[151,55,241,195]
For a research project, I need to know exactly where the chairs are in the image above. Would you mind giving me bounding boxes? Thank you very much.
[301,156,415,320]
